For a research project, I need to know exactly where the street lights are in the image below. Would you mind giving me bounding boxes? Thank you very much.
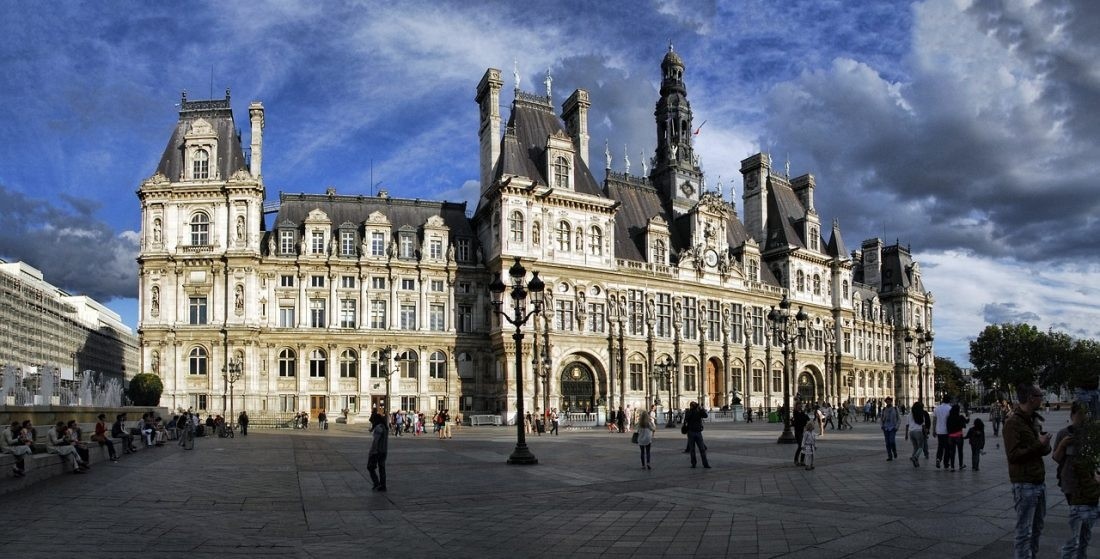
[487,258,547,465]
[769,293,807,445]
[907,323,934,413]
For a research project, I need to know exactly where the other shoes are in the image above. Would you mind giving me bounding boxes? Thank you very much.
[704,447,708,450]
[910,455,921,468]
[793,460,815,471]
[393,429,453,439]
[373,484,388,492]
[75,468,84,474]
[704,466,712,469]
[647,464,651,470]
[146,437,195,447]
[689,465,696,468]
[683,450,689,454]
[886,453,897,461]
[125,445,137,455]
[111,455,120,461]
[817,425,855,439]
[642,466,645,470]
[925,454,981,472]
[80,461,90,470]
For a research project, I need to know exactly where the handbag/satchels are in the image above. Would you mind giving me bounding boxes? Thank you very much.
[91,434,106,442]
[631,432,639,444]
[680,423,688,434]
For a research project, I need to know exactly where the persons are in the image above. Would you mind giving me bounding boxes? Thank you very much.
[1045,401,1050,414]
[920,402,931,459]
[989,399,1002,437]
[1001,399,1012,425]
[801,421,818,471]
[1052,399,1100,559]
[686,401,712,469]
[634,410,656,471]
[964,399,970,417]
[0,405,249,476]
[932,394,952,469]
[366,413,389,492]
[792,400,810,467]
[962,418,985,471]
[880,396,901,461]
[293,397,905,442]
[903,402,926,467]
[685,407,707,453]
[946,404,970,471]
[999,384,1054,559]
